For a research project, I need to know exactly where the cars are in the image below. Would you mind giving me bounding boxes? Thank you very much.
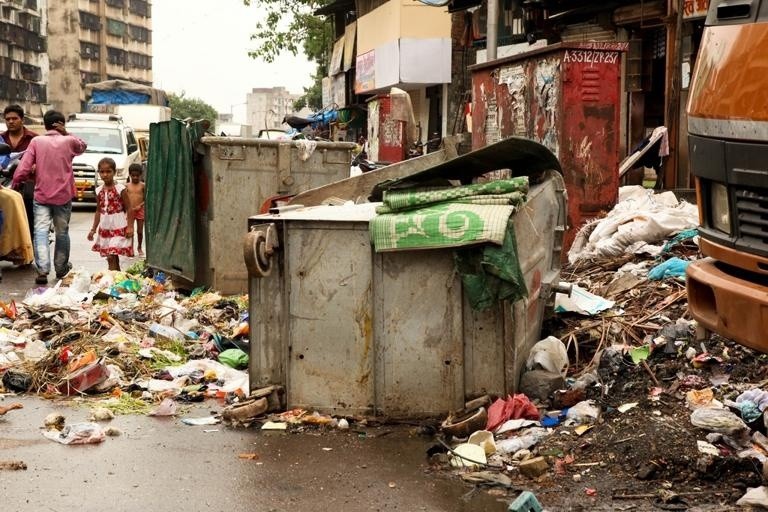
[64,112,142,208]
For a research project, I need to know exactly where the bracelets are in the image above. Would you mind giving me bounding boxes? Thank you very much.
[91,229,96,233]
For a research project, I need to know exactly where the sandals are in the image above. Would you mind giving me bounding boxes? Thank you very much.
[36,274,48,285]
[56,262,72,278]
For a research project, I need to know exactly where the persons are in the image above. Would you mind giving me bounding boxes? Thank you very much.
[123,164,145,256]
[86,156,135,272]
[11,110,88,285]
[0,105,39,151]
